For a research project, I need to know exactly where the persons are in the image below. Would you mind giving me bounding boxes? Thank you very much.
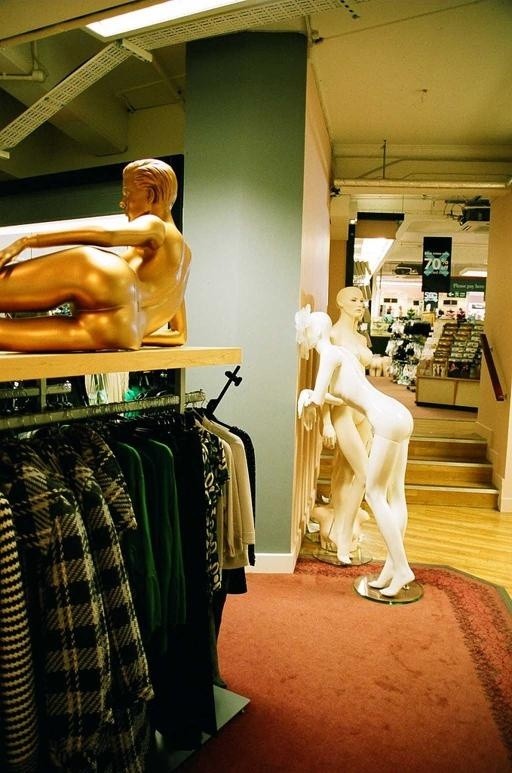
[326,286,373,566]
[297,310,416,598]
[0,156,193,353]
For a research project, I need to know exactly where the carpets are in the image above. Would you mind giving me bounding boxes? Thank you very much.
[177,554,511,771]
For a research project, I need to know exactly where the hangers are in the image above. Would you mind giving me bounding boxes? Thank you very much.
[0,383,225,463]
